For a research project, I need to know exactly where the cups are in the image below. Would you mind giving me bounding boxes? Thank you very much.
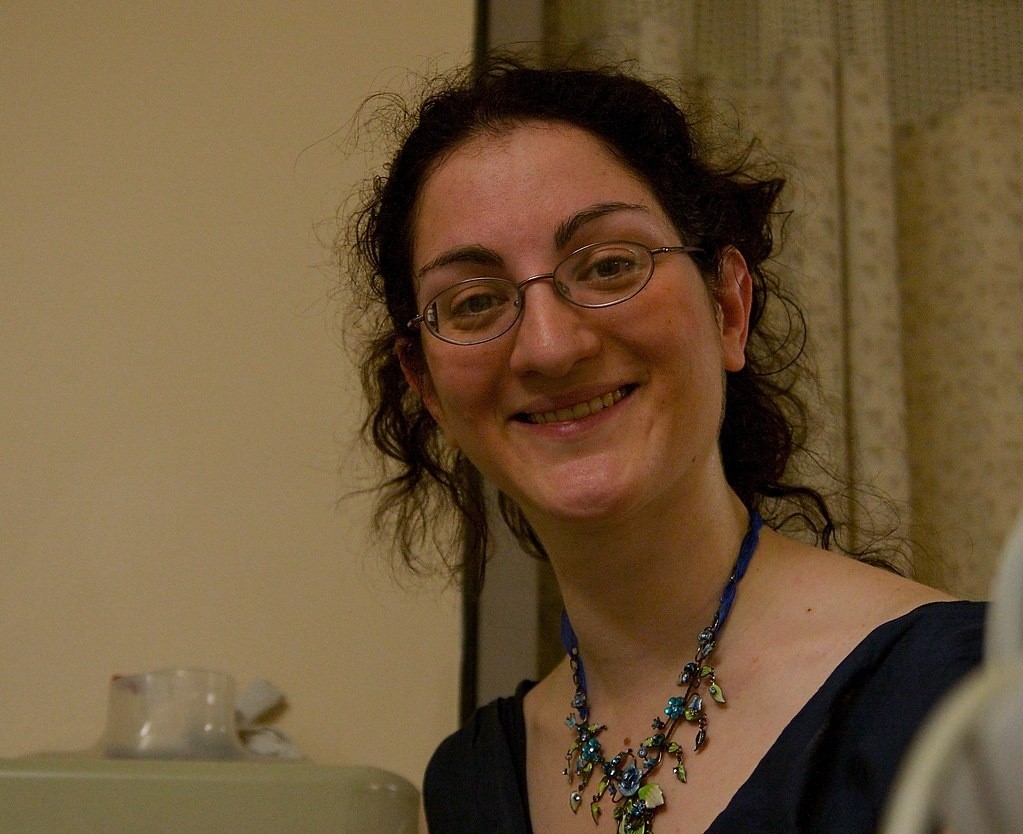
[107,668,236,761]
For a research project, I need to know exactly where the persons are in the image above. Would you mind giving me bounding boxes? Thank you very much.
[372,67,992,834]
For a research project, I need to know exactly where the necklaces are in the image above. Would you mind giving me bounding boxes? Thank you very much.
[561,508,766,834]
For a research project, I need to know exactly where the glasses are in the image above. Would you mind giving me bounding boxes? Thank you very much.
[407,239,706,345]
